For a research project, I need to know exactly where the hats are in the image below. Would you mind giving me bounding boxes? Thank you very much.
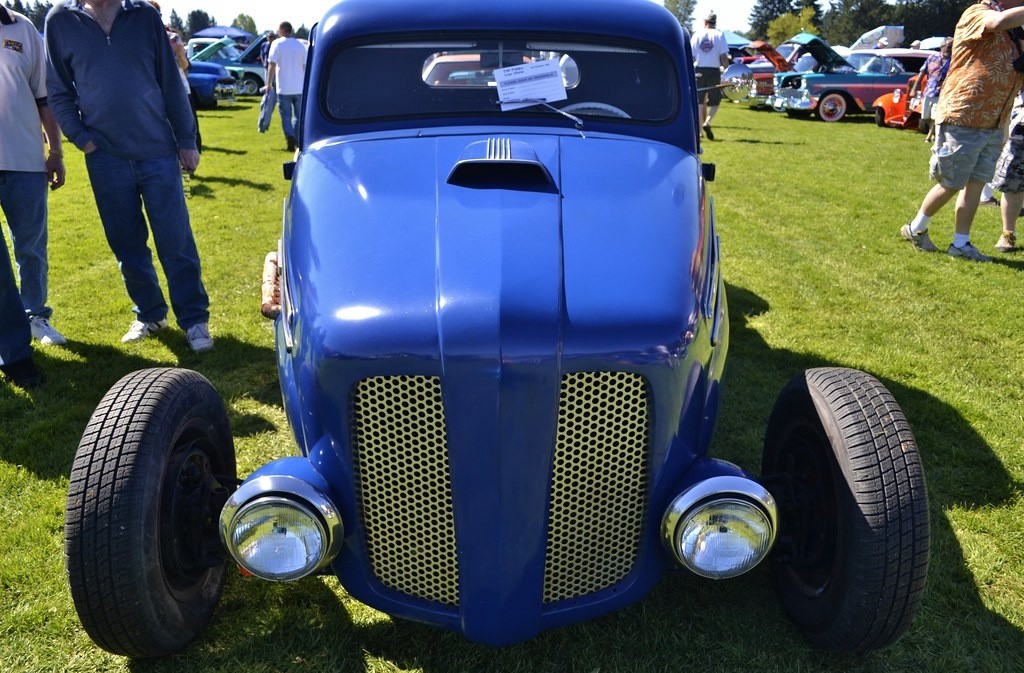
[704,13,716,22]
[911,40,921,47]
[879,37,888,45]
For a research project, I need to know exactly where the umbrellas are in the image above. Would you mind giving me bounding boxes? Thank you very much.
[919,37,946,50]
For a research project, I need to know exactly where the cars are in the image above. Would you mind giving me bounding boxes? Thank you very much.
[723,38,851,114]
[421,55,534,86]
[180,35,275,111]
[60,0,930,656]
[766,32,940,124]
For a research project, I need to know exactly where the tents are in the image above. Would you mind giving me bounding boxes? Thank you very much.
[723,31,756,57]
[193,26,254,46]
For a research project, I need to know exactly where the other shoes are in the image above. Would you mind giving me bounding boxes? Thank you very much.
[288,136,295,153]
[703,123,713,140]
[925,139,931,142]
[1019,208,1024,216]
[979,196,1000,207]
[5,362,48,391]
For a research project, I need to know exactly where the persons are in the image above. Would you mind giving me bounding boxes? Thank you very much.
[872,37,888,49]
[145,0,202,177]
[0,1,65,346]
[690,13,729,139]
[43,0,212,351]
[900,0,1024,260]
[265,21,307,151]
[260,32,276,87]
[0,221,41,391]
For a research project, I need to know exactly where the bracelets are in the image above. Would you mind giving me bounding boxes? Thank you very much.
[49,149,64,158]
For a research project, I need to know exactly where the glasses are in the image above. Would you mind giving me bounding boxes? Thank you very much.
[269,36,274,38]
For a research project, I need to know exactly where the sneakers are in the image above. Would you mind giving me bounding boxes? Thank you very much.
[995,231,1016,252]
[29,315,66,345]
[186,323,214,353]
[121,314,168,343]
[946,242,996,262]
[900,221,939,253]
